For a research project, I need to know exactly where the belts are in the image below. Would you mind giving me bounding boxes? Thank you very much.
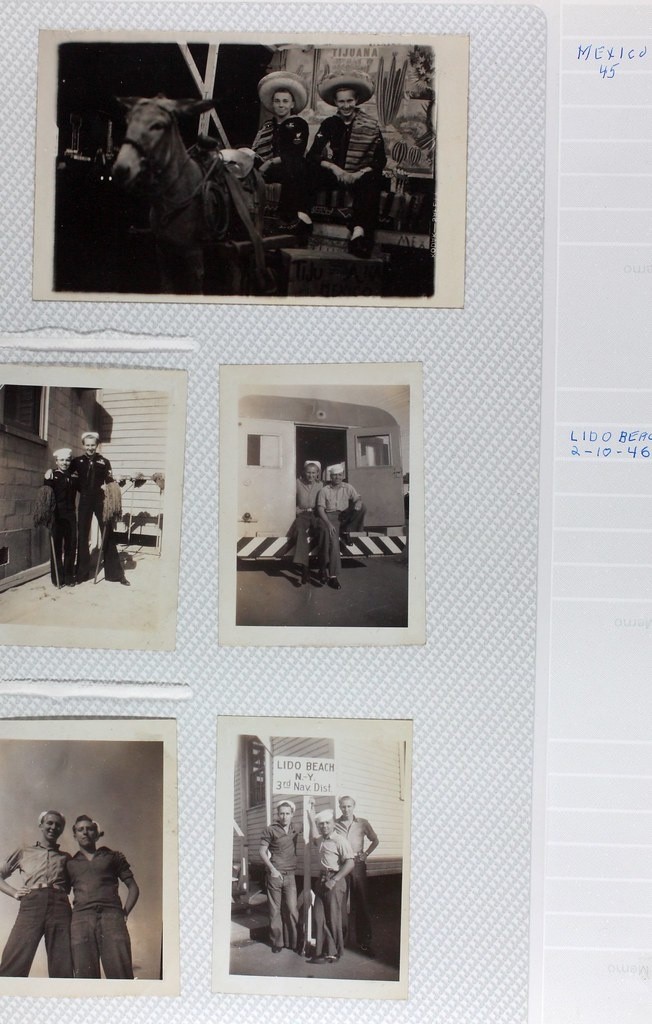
[30,884,66,891]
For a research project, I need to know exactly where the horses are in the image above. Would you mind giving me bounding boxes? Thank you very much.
[110,95,274,296]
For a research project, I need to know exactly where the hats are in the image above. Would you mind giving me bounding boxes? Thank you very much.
[313,809,334,824]
[258,71,309,115]
[52,448,72,458]
[38,810,66,828]
[82,432,99,440]
[317,71,375,107]
[91,821,105,842]
[328,464,343,475]
[277,800,296,812]
[304,460,321,470]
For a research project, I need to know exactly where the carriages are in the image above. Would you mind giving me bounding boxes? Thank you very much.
[109,41,435,297]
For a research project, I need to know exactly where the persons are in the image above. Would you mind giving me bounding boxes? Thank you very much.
[64,814,141,980]
[331,796,380,959]
[316,463,366,588]
[0,809,74,977]
[45,431,130,586]
[277,73,386,260]
[306,806,355,965]
[259,799,302,954]
[43,447,108,586]
[248,73,310,236]
[294,459,330,584]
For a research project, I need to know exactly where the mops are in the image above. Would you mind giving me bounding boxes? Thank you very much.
[111,474,132,486]
[151,471,165,549]
[93,482,123,583]
[31,486,62,590]
[127,471,145,546]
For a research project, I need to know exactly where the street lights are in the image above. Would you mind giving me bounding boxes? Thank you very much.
[252,740,274,825]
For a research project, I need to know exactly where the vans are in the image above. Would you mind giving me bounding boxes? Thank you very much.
[233,393,408,562]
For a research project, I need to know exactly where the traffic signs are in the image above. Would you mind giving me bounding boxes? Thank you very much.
[272,756,338,798]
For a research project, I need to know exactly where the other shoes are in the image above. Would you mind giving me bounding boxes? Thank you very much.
[272,946,282,953]
[301,569,310,584]
[329,577,341,589]
[361,944,375,957]
[293,949,301,953]
[75,576,88,584]
[348,235,371,258]
[279,218,313,235]
[341,534,353,546]
[319,570,327,584]
[310,953,336,964]
[120,577,130,586]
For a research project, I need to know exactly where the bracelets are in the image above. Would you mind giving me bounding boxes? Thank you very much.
[330,875,337,884]
[359,170,365,176]
[365,851,369,857]
[269,158,274,166]
